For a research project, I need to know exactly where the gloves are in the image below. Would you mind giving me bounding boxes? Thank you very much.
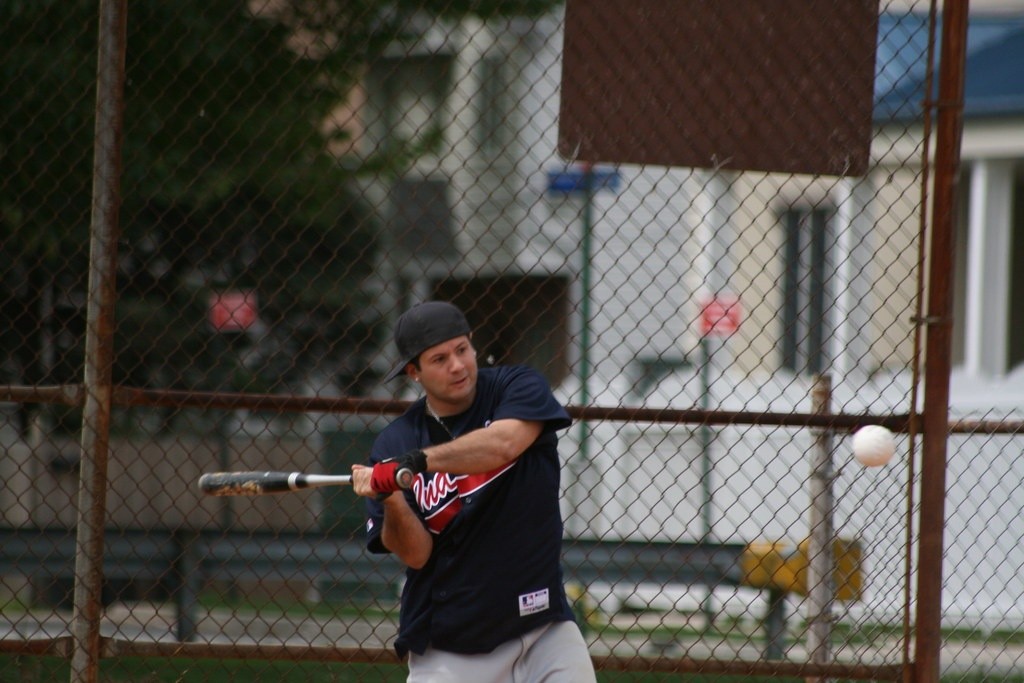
[370,449,427,493]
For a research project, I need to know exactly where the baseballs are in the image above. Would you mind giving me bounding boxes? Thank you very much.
[849,425,896,467]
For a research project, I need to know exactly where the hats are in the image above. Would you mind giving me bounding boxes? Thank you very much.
[382,301,471,383]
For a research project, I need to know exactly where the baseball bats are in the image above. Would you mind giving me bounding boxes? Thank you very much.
[197,468,415,498]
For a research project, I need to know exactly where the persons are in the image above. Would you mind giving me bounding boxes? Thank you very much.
[351,301,597,683]
